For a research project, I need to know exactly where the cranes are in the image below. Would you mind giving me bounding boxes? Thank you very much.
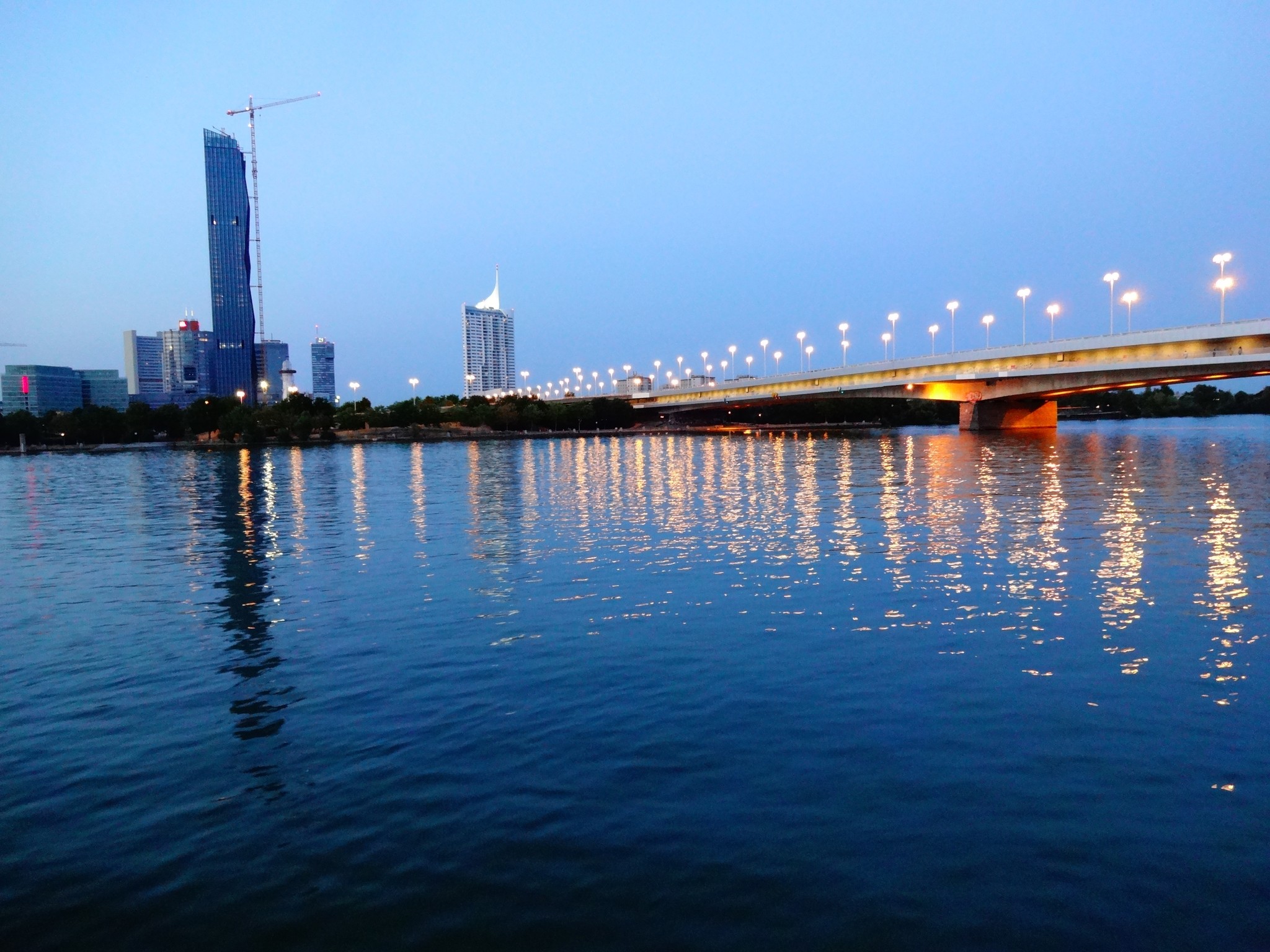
[226,88,320,406]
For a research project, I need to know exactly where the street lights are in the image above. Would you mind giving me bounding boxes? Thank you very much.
[929,325,939,355]
[350,381,359,410]
[634,378,641,392]
[883,312,899,362]
[464,374,477,395]
[722,345,736,383]
[839,322,849,367]
[982,314,994,348]
[1211,252,1236,324]
[773,351,782,375]
[408,377,420,406]
[1101,271,1138,337]
[797,331,813,373]
[650,352,713,391]
[760,340,768,378]
[746,356,752,380]
[586,365,631,395]
[1017,287,1032,345]
[518,367,583,399]
[946,301,959,353]
[1045,304,1060,341]
